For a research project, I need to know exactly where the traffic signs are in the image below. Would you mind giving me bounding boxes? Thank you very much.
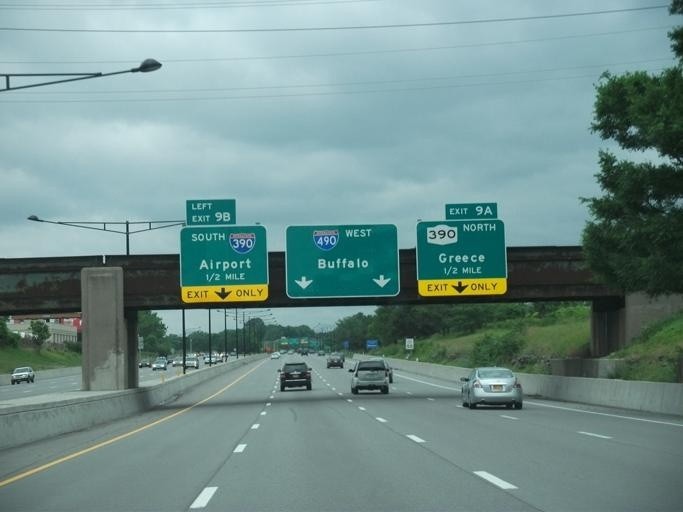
[287,225,400,299]
[447,203,499,218]
[418,222,508,297]
[186,202,236,224]
[180,225,269,303]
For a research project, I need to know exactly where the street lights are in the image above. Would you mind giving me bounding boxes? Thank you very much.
[0,59,161,93]
[26,216,185,255]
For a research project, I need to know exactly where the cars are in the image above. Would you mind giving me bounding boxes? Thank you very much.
[271,350,287,359]
[288,348,295,354]
[460,367,523,410]
[139,359,151,368]
[11,367,35,384]
[278,363,311,390]
[327,352,344,368]
[349,358,393,394]
[231,352,236,356]
[203,352,228,363]
[152,361,167,370]
[319,350,325,356]
[167,354,199,369]
[298,347,315,356]
